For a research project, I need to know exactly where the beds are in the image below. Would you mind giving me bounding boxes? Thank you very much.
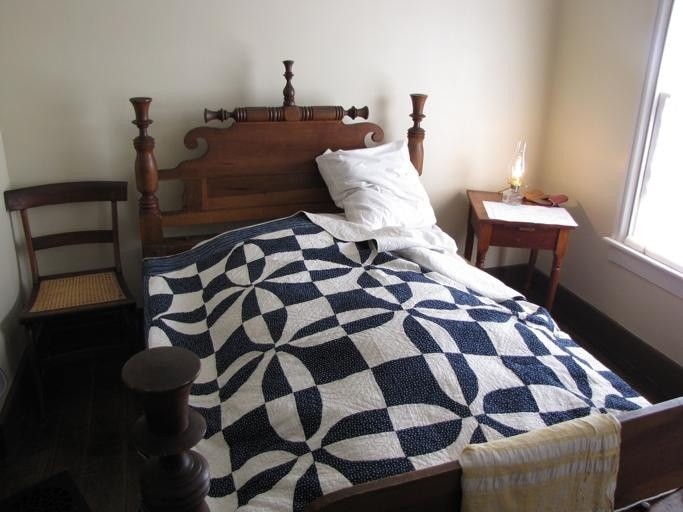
[121,57,682,511]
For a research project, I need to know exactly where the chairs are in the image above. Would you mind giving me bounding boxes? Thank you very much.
[2,180,141,421]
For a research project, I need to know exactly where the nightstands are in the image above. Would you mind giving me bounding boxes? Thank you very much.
[463,187,580,319]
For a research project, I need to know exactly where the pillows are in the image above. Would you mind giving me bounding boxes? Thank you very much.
[313,135,438,238]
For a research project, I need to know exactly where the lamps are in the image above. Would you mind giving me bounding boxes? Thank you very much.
[500,138,526,206]
